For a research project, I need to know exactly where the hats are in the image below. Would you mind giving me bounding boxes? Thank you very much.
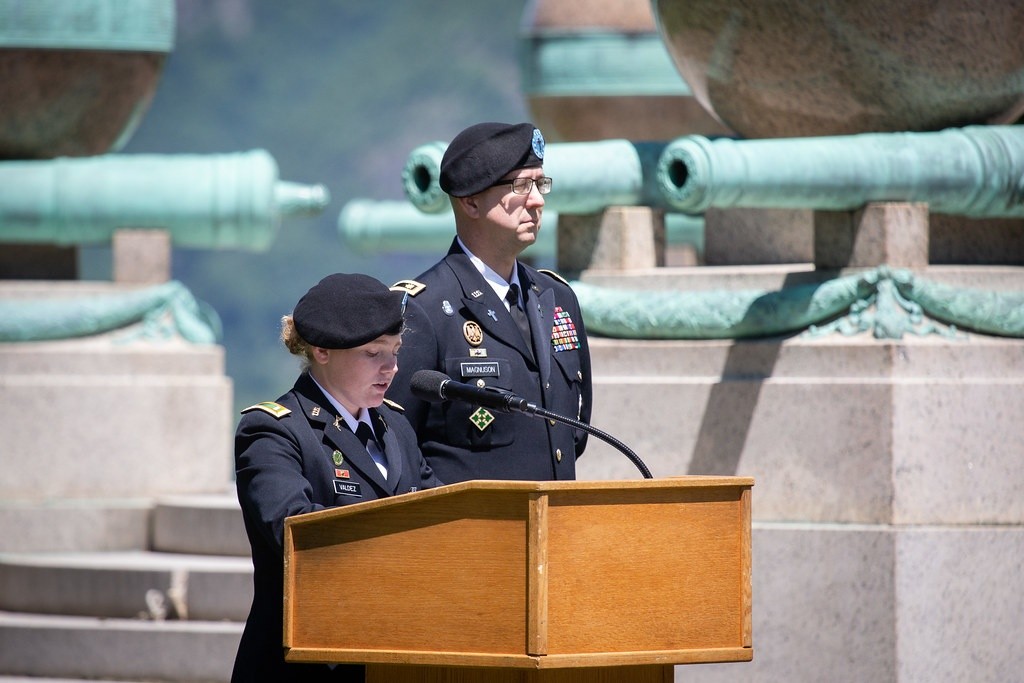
[292,273,408,349]
[439,122,545,198]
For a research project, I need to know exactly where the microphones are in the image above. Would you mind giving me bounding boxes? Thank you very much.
[409,370,528,413]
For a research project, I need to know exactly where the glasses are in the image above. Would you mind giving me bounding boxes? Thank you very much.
[491,177,552,195]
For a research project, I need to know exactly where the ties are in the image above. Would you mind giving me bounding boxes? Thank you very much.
[506,283,538,367]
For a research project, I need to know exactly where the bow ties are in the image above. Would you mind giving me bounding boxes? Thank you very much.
[355,421,382,454]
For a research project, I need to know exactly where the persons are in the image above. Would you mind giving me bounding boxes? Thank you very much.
[389,124,593,484]
[229,274,447,683]
